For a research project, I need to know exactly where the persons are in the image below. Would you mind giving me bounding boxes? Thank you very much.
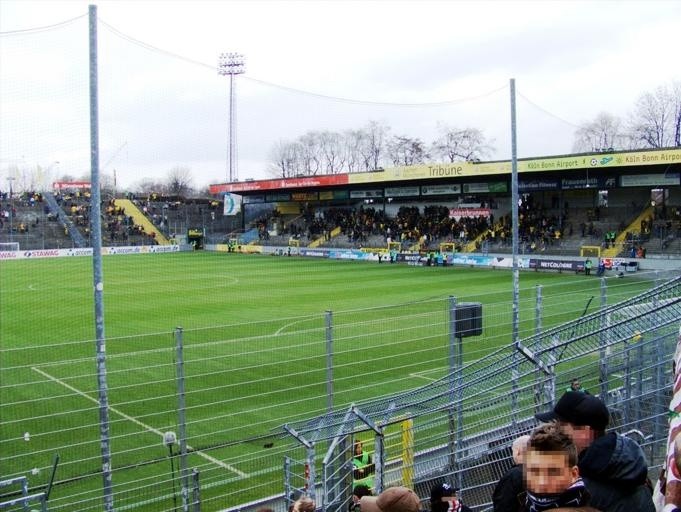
[255,484,473,512]
[351,441,372,488]
[490,431,530,512]
[516,425,595,512]
[566,377,588,393]
[534,391,657,512]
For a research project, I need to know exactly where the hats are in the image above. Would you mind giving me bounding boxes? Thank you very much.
[536,391,609,429]
[351,485,371,499]
[431,482,459,499]
[361,487,422,512]
